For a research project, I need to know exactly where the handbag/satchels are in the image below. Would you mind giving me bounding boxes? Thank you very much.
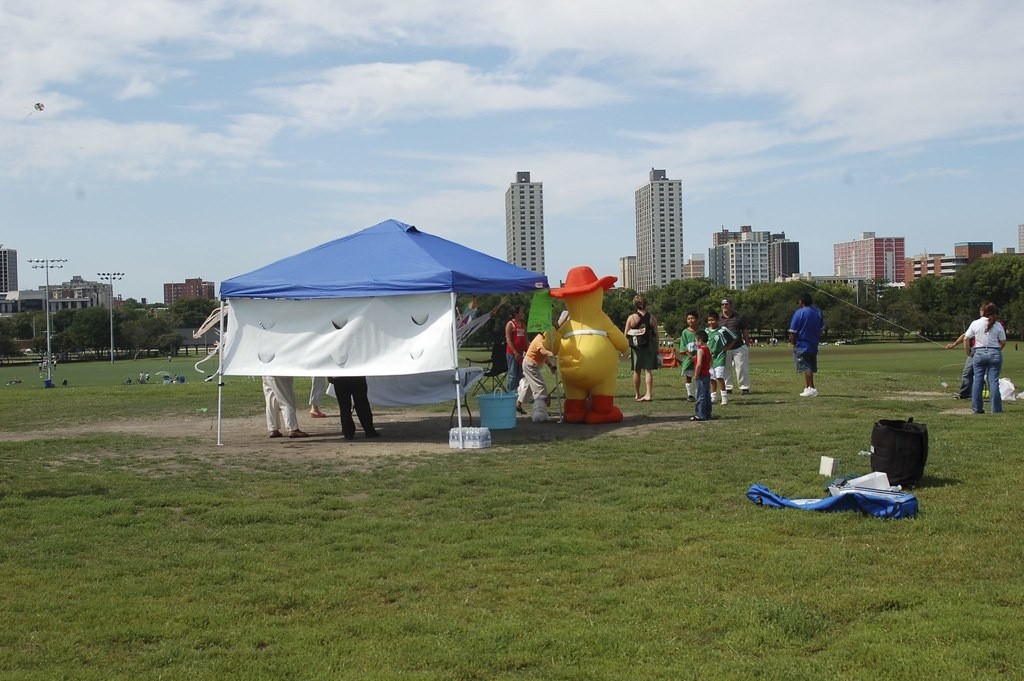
[870,417,928,489]
[998,376,1016,401]
[627,314,649,349]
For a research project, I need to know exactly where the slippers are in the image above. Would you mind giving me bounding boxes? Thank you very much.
[636,397,652,402]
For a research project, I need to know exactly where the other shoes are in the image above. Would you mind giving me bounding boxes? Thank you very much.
[366,430,381,438]
[690,415,705,422]
[287,429,309,438]
[269,430,283,438]
[310,411,326,418]
[516,406,528,414]
[345,435,353,440]
[687,395,695,402]
[724,390,733,395]
[741,389,749,395]
[954,394,969,400]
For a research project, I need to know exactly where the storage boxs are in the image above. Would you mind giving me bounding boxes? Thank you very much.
[477,393,520,429]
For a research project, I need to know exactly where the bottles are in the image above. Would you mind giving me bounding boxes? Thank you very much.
[449,426,491,441]
[458,354,470,368]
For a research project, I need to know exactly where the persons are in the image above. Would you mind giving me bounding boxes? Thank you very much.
[964,302,1006,414]
[39,360,57,370]
[516,321,559,423]
[308,377,327,418]
[261,376,309,437]
[624,296,660,402]
[127,372,185,384]
[680,311,704,402]
[789,295,824,397]
[660,332,674,348]
[947,307,1005,401]
[766,337,778,346]
[328,377,380,439]
[689,330,712,420]
[506,306,529,393]
[718,299,750,395]
[168,355,172,361]
[705,312,738,405]
[750,338,759,346]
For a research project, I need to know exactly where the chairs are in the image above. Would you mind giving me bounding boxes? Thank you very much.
[465,357,508,397]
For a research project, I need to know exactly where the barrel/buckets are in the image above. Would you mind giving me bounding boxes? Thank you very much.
[179,376,185,382]
[476,387,519,429]
[44,379,51,387]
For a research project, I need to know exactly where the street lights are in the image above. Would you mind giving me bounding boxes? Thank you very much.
[96,272,124,363]
[27,258,68,381]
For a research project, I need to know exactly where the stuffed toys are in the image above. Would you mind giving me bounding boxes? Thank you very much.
[549,266,629,424]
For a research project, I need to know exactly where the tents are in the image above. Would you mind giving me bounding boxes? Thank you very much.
[217,220,563,446]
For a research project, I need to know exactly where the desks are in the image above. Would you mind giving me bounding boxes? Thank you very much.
[326,366,484,428]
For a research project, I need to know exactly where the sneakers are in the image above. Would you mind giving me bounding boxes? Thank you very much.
[798,387,819,397]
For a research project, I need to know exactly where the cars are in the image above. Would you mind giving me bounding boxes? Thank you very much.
[25,348,36,354]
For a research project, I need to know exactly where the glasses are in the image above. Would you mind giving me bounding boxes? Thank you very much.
[721,303,730,307]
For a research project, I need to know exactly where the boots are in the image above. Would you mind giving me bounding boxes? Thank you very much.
[720,390,728,406]
[710,392,717,404]
